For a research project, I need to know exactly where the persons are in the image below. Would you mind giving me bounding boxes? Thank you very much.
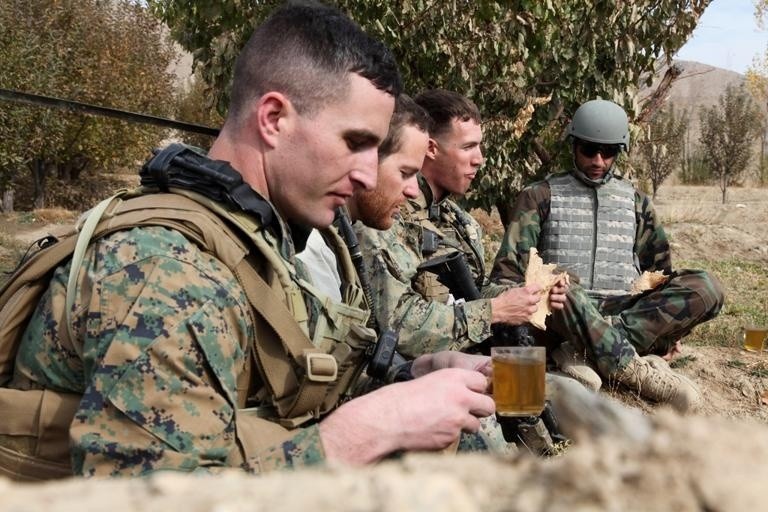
[394,85,654,450]
[1,0,499,488]
[330,86,564,460]
[483,97,725,418]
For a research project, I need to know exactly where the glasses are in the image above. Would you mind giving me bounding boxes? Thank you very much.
[572,136,621,159]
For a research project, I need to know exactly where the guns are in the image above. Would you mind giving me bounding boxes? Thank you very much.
[416,250,558,457]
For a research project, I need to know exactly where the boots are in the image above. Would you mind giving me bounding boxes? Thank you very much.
[608,348,704,415]
[550,315,613,392]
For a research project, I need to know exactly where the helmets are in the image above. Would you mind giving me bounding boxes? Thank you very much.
[571,99,631,153]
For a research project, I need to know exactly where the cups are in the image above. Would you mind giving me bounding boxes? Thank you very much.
[737,324,768,352]
[490,346,546,418]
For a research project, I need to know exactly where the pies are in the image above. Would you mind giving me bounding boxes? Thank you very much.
[524,246,572,331]
[630,269,672,296]
